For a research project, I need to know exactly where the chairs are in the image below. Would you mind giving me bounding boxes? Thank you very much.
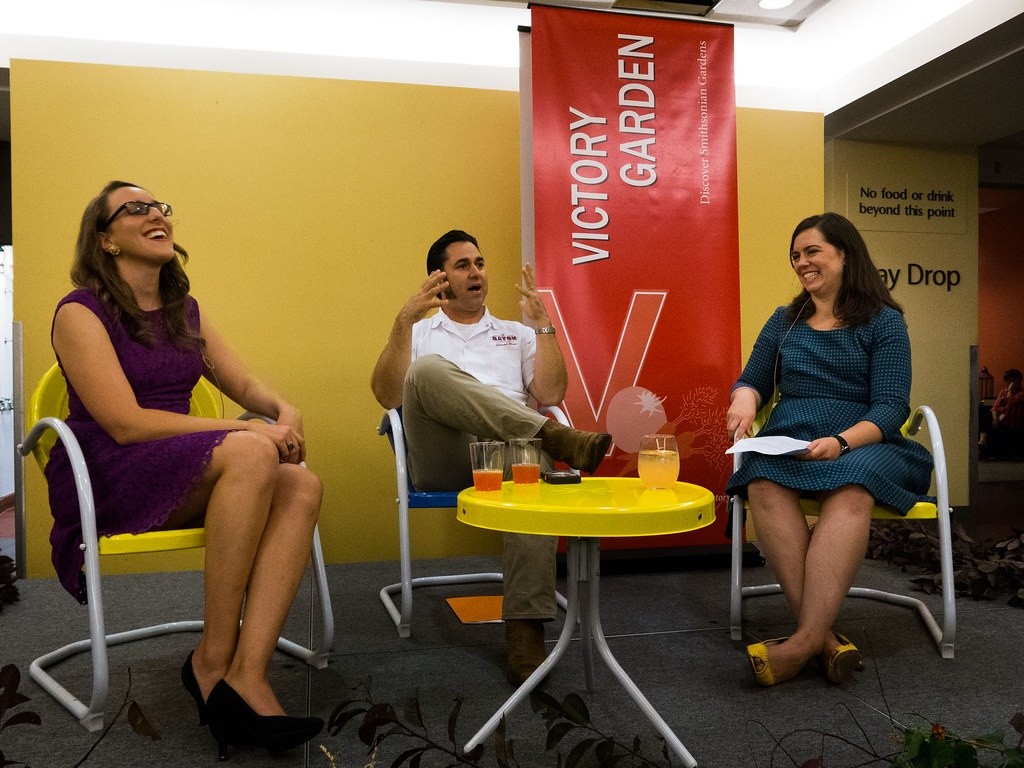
[18,360,332,732]
[727,404,954,659]
[372,404,582,637]
[988,401,1023,460]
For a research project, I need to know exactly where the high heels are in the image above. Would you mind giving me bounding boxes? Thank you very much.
[827,631,865,687]
[746,635,793,686]
[181,649,323,761]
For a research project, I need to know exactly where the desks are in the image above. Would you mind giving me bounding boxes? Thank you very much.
[448,476,720,767]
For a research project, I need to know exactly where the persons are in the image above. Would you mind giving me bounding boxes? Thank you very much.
[725,212,933,689]
[978,368,1024,448]
[372,231,611,685]
[46,181,323,761]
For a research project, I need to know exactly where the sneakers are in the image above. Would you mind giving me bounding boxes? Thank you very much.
[506,617,551,687]
[530,418,612,475]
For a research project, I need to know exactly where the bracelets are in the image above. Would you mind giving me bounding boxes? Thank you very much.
[535,325,556,335]
[1007,389,1012,392]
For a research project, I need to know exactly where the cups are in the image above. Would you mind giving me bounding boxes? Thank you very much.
[509,437,542,483]
[637,433,680,489]
[469,441,505,491]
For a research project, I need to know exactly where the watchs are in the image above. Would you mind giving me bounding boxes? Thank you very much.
[830,434,851,457]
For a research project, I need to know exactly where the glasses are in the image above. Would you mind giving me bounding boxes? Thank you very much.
[101,200,174,233]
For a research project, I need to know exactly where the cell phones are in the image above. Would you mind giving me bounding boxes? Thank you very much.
[541,471,581,484]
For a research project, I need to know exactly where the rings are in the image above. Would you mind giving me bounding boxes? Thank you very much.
[287,442,295,451]
